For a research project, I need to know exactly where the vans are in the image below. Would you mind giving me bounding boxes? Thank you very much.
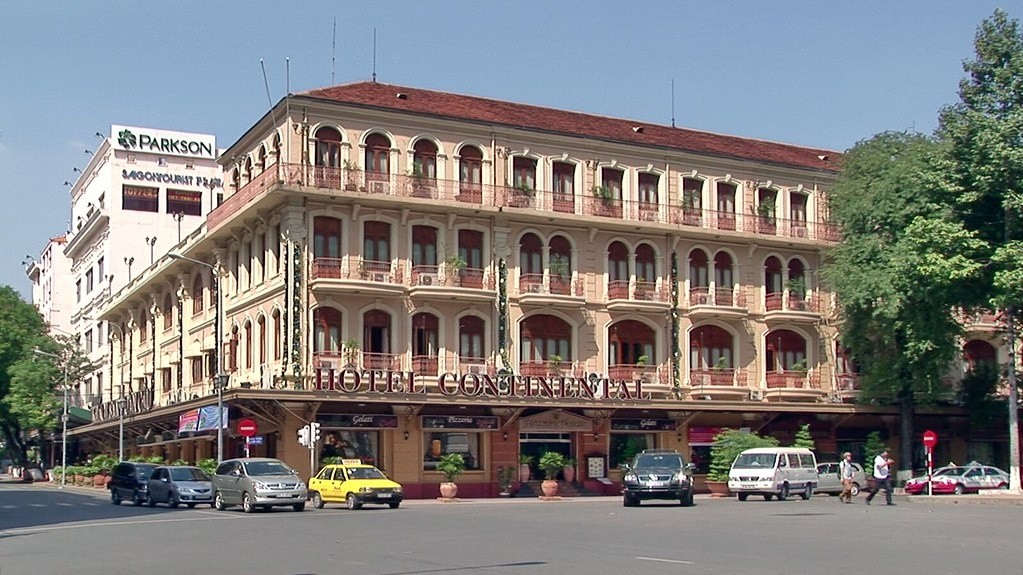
[728,446,820,502]
[814,462,868,497]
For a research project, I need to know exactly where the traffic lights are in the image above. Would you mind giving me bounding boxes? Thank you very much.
[311,422,320,443]
[297,425,310,446]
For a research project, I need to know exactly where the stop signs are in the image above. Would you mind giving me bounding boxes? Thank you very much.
[238,420,256,437]
[922,430,938,447]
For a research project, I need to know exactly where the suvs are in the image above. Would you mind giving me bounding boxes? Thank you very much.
[620,448,697,507]
[211,456,308,513]
[107,461,163,507]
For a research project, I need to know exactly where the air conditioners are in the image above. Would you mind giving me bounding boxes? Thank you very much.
[692,293,714,305]
[525,283,545,294]
[465,364,487,375]
[582,371,604,395]
[369,272,389,283]
[417,272,439,286]
[748,389,764,400]
[791,227,809,239]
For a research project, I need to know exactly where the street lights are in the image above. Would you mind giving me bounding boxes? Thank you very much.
[81,314,123,465]
[167,252,223,465]
[34,348,68,486]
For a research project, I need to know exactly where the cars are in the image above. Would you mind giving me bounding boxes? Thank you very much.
[308,458,404,511]
[146,465,212,510]
[904,460,1011,495]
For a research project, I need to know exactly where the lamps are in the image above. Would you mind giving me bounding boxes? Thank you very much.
[21,255,34,266]
[63,132,105,188]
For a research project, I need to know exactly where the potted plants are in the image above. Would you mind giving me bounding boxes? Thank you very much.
[437,454,464,502]
[520,454,533,482]
[52,455,112,488]
[343,159,360,190]
[537,451,578,500]
[549,354,562,378]
[591,184,617,215]
[714,356,726,380]
[343,339,359,373]
[758,195,776,233]
[633,354,648,383]
[785,276,805,309]
[408,163,430,197]
[865,431,889,491]
[792,358,807,387]
[496,464,514,496]
[442,242,466,287]
[681,188,700,225]
[512,182,531,206]
[704,431,733,496]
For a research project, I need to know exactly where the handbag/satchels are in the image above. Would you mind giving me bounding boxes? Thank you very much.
[837,461,845,479]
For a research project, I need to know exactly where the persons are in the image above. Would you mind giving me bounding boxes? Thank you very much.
[838,452,855,504]
[865,447,897,505]
